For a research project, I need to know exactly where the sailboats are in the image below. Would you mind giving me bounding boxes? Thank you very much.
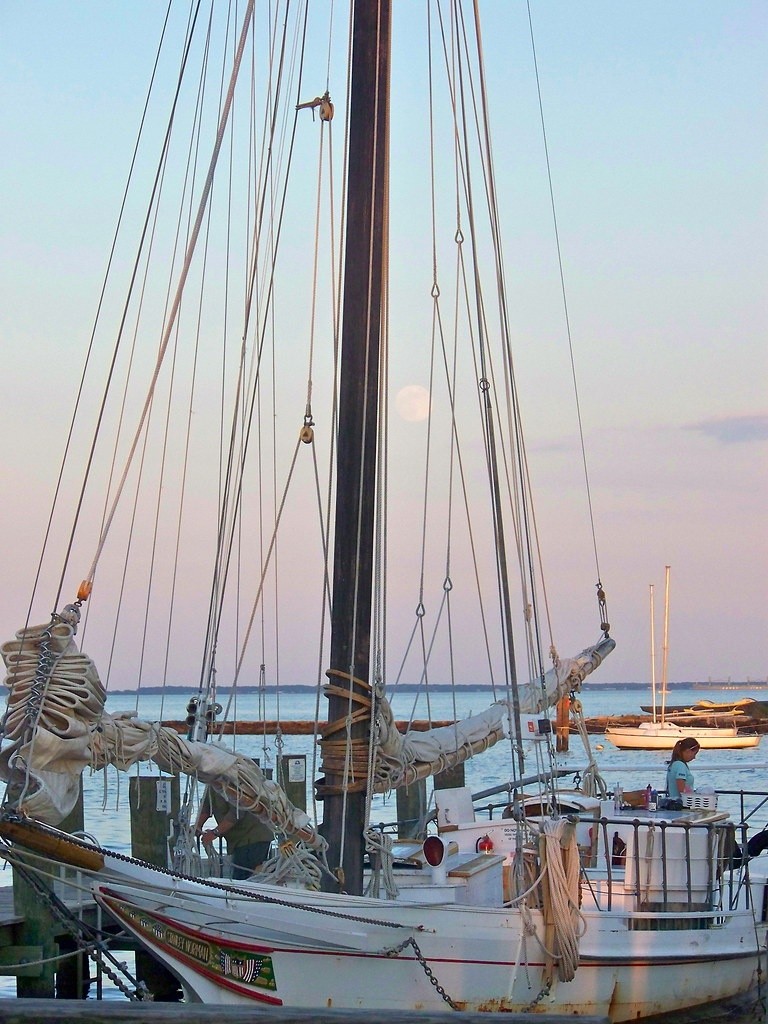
[0,1,768,1024]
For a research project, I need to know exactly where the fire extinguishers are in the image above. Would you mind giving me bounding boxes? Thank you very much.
[475,829,496,855]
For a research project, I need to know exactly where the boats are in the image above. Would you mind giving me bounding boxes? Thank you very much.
[603,715,762,753]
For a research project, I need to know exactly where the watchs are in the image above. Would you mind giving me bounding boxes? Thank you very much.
[213,829,220,838]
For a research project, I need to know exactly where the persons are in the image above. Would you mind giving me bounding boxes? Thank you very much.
[666,737,700,802]
[195,784,276,881]
